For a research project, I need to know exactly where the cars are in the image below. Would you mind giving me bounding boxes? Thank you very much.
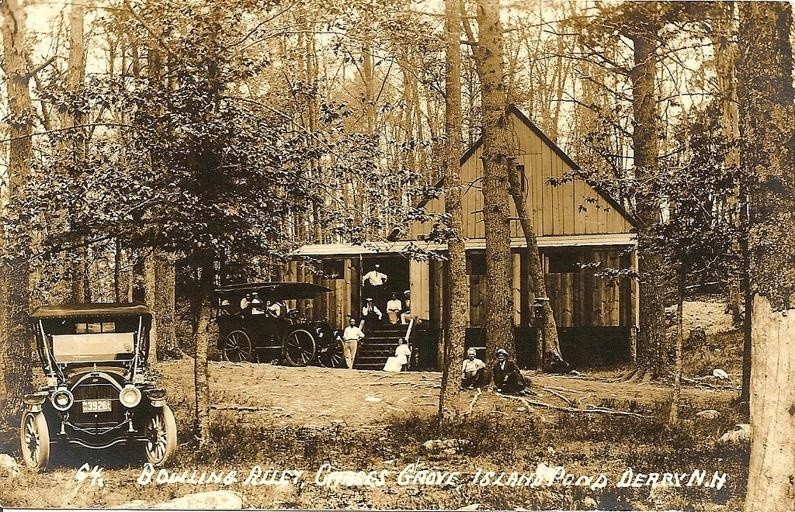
[214,281,345,368]
[20,300,177,473]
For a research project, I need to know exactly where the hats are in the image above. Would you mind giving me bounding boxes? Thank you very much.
[496,349,510,357]
[404,289,410,296]
[365,297,373,300]
[375,264,380,267]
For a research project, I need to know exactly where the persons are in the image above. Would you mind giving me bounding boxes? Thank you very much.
[396,289,410,325]
[362,264,388,307]
[386,291,402,325]
[461,347,486,390]
[341,318,364,369]
[493,348,526,394]
[359,297,382,334]
[382,338,412,372]
[221,291,286,321]
